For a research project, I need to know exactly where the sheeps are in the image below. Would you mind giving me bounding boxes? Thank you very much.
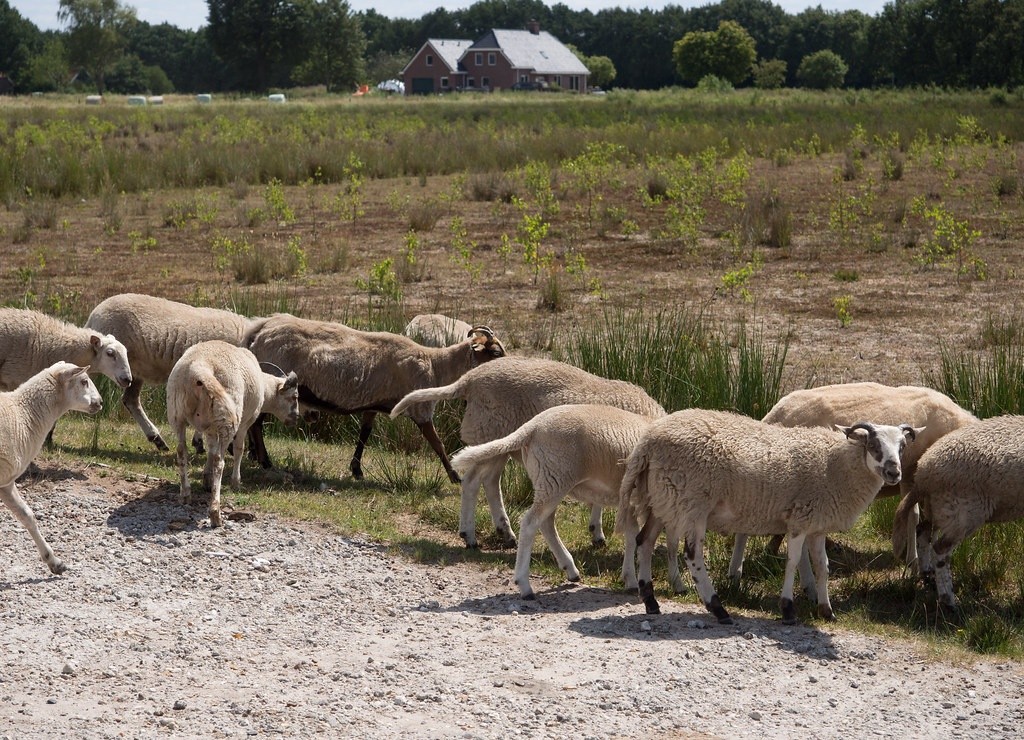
[0,306,133,575]
[81,293,1024,633]
